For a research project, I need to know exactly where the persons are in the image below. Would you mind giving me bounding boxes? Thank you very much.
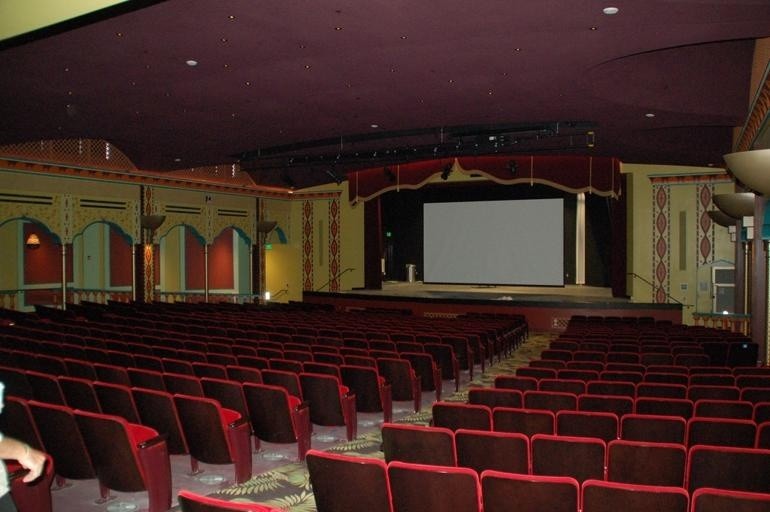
[0,379,48,511]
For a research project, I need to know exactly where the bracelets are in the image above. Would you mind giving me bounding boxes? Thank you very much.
[20,438,33,463]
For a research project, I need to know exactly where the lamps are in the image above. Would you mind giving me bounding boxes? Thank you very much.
[508,160,516,176]
[722,148,770,197]
[326,168,344,185]
[712,192,755,241]
[382,167,396,183]
[257,221,277,245]
[707,210,737,241]
[441,163,453,180]
[140,215,167,244]
[26,233,42,248]
